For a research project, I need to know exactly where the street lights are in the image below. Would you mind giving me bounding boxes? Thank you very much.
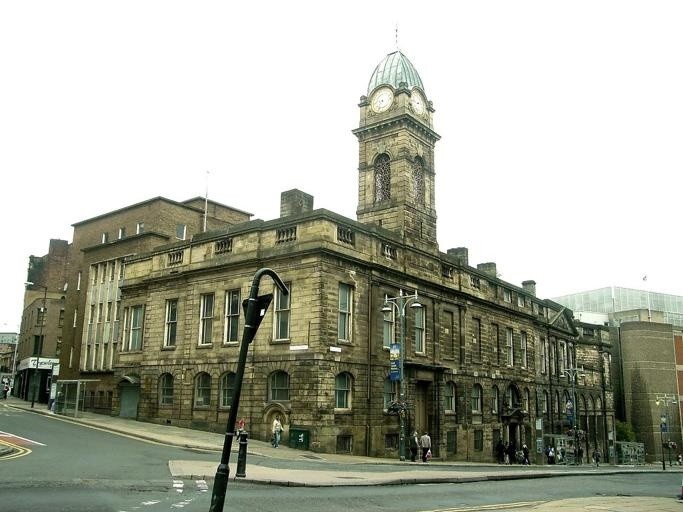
[559,363,585,468]
[380,288,423,463]
[655,393,678,467]
[24,280,48,407]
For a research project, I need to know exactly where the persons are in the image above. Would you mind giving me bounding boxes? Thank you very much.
[3,383,9,399]
[272,414,284,448]
[677,453,682,465]
[419,432,432,462]
[496,439,601,468]
[236,416,246,442]
[408,431,420,461]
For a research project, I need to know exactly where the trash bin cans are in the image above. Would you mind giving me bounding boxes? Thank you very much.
[288,428,309,451]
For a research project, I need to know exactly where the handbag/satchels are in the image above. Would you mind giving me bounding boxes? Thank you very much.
[270,435,276,446]
[424,449,432,458]
